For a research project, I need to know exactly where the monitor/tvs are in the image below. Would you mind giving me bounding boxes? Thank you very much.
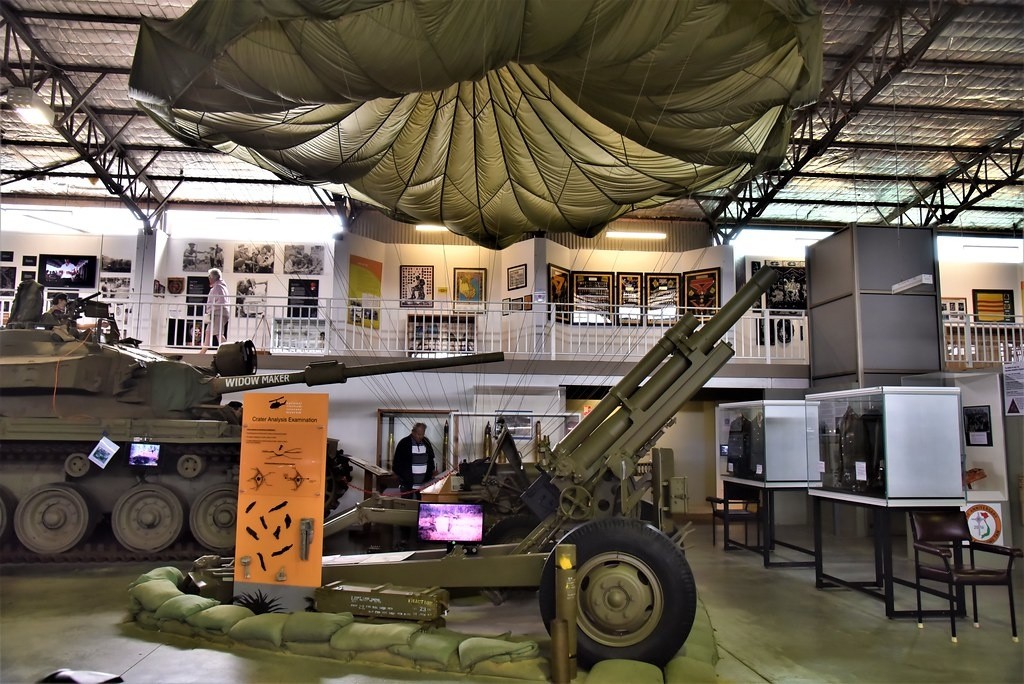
[129,443,161,467]
[37,254,97,289]
[416,502,484,553]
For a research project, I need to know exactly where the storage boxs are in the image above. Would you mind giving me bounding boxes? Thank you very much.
[805,386,968,500]
[717,400,821,481]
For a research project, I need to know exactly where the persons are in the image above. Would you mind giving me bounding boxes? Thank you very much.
[58,258,77,287]
[285,252,322,275]
[392,423,436,500]
[428,513,437,532]
[184,243,222,268]
[234,244,274,273]
[102,281,107,297]
[200,268,230,353]
[45,293,109,330]
[236,279,256,315]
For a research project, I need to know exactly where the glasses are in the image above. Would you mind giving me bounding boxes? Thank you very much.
[64,298,68,303]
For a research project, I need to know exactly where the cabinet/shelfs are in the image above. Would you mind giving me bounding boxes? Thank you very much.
[269,318,328,350]
[405,311,478,357]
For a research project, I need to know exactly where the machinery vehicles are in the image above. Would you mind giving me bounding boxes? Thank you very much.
[180,264,781,671]
[0,280,504,563]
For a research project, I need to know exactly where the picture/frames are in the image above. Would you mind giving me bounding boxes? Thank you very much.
[453,267,488,315]
[501,298,511,316]
[940,297,967,323]
[963,405,993,447]
[399,264,435,307]
[683,267,720,324]
[571,271,615,326]
[507,263,527,290]
[548,263,570,324]
[645,273,681,326]
[523,294,532,310]
[617,271,643,327]
[512,297,523,311]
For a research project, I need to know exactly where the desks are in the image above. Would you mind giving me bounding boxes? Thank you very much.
[720,475,823,573]
[805,488,968,623]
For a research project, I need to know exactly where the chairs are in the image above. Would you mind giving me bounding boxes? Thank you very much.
[706,492,763,552]
[910,509,1020,643]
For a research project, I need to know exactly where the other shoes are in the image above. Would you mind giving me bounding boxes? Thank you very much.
[400,540,408,548]
[415,541,424,549]
